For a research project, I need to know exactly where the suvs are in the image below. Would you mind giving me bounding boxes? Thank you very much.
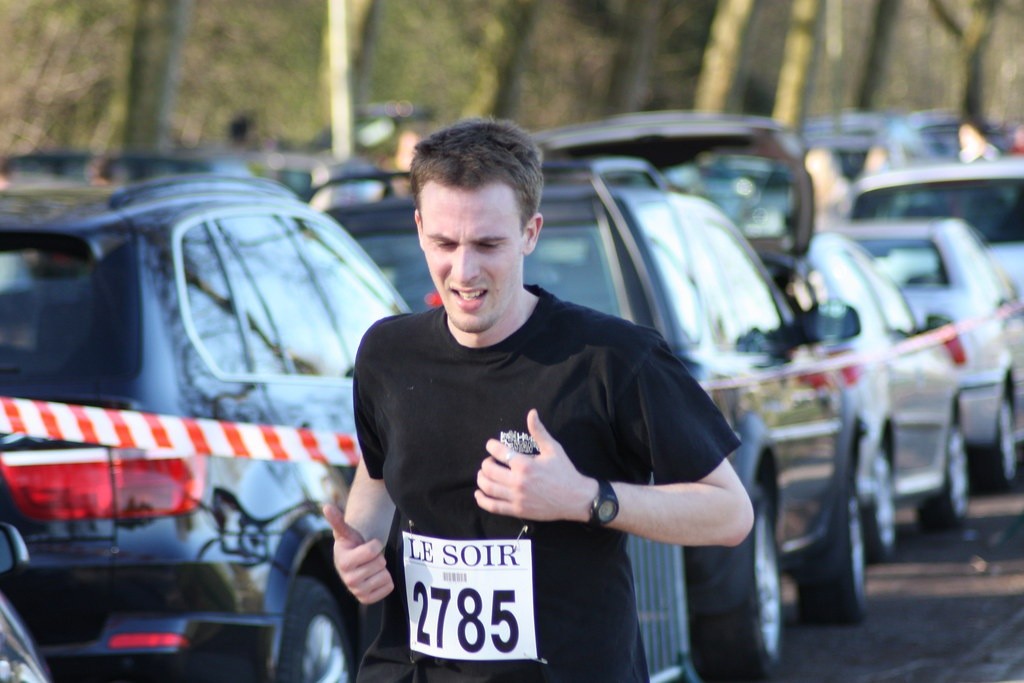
[0,151,418,681]
[297,159,867,681]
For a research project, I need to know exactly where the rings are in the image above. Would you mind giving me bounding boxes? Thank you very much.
[505,451,515,464]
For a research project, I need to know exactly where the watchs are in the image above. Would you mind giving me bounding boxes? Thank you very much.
[586,475,620,531]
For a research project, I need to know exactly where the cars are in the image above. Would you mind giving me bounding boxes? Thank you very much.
[524,103,1024,568]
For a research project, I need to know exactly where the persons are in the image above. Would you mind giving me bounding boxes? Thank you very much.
[957,115,1002,165]
[323,115,754,683]
